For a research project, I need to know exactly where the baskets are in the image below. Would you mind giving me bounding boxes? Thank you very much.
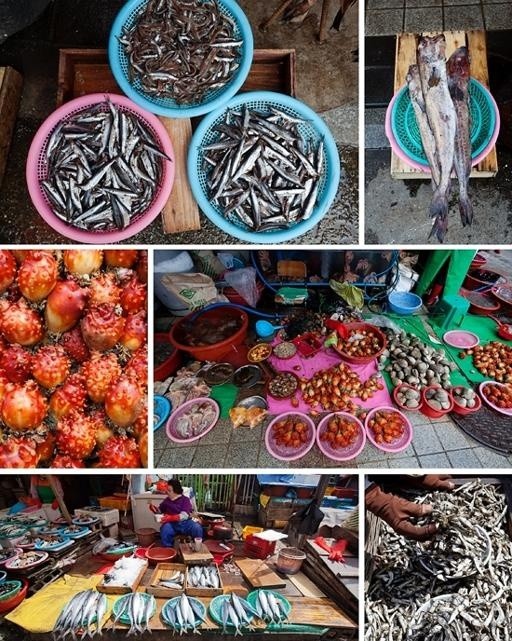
[0,514,100,613]
[26,92,176,244]
[108,0,254,119]
[342,505,358,532]
[99,496,130,511]
[244,535,276,560]
[384,76,501,174]
[187,91,342,244]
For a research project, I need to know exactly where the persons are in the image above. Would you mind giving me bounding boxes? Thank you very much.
[30,474,64,510]
[149,479,203,548]
[365,474,455,543]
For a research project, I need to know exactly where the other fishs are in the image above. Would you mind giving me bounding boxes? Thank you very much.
[157,564,220,589]
[114,0,245,105]
[406,64,448,243]
[52,588,292,640]
[363,475,512,641]
[40,101,174,235]
[445,46,475,227]
[415,32,458,221]
[198,100,326,233]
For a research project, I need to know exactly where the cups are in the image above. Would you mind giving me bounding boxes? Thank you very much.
[153,514,164,524]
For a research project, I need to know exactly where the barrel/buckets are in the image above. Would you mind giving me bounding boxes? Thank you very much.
[137,527,155,547]
[278,548,307,574]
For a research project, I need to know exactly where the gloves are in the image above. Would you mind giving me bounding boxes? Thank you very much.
[365,484,440,540]
[399,474,455,490]
[314,536,349,562]
[161,514,181,523]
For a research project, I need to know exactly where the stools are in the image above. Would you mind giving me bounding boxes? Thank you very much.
[431,293,471,330]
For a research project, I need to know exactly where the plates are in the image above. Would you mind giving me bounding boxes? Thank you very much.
[235,393,269,429]
[204,364,263,389]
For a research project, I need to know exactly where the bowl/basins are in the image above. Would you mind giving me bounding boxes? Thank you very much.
[317,410,367,462]
[203,516,226,527]
[95,539,238,564]
[264,412,317,462]
[165,397,220,444]
[464,254,512,316]
[388,291,423,314]
[332,320,386,365]
[60,588,290,628]
[0,511,98,617]
[269,484,315,500]
[154,395,170,430]
[220,272,266,304]
[416,523,491,579]
[394,379,512,417]
[364,405,414,453]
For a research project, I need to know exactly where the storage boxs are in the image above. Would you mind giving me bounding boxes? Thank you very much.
[74,495,133,539]
[240,524,276,559]
